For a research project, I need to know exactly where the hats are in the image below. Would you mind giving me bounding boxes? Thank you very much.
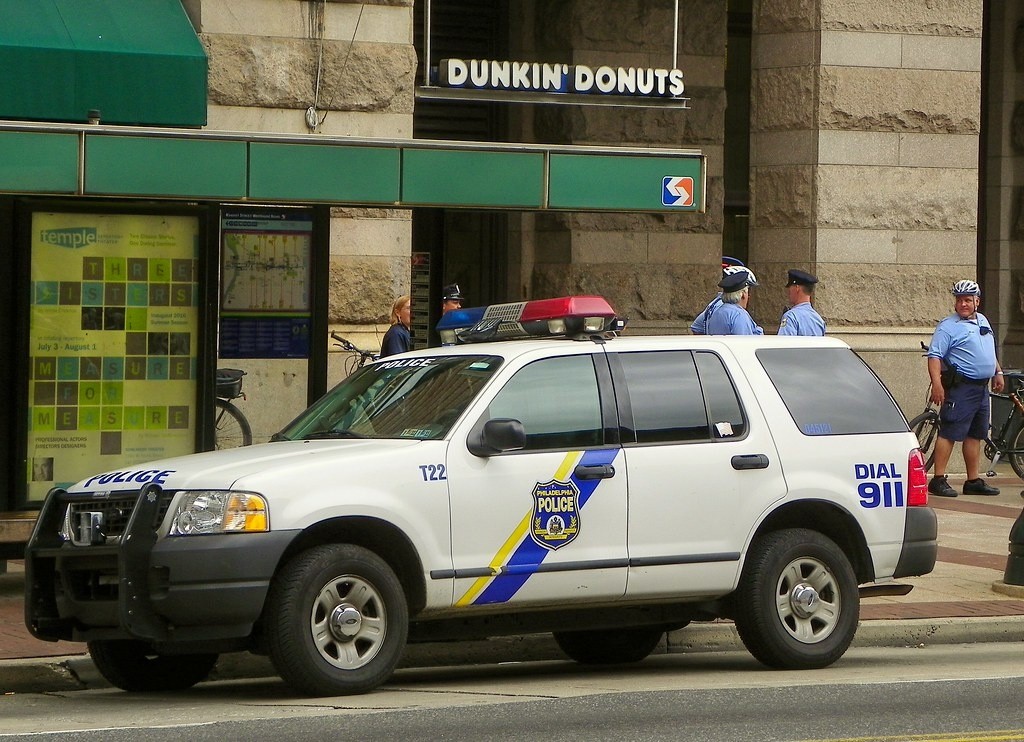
[718,272,748,293]
[442,283,464,300]
[785,270,818,287]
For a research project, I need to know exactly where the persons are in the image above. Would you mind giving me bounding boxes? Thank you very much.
[691,257,764,335]
[926,280,1004,497]
[777,269,826,336]
[441,284,463,343]
[380,296,411,359]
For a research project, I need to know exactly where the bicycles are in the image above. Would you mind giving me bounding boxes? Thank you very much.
[328,329,410,431]
[906,341,1024,485]
[215,367,254,449]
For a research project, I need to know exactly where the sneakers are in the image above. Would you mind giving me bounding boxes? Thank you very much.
[963,479,1000,495]
[928,475,958,497]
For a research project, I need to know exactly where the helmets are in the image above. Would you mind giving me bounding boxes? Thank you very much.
[723,266,758,288]
[952,280,981,296]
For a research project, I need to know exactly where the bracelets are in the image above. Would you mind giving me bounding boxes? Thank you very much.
[996,372,1003,375]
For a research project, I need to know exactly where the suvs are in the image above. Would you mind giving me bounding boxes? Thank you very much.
[21,296,939,698]
